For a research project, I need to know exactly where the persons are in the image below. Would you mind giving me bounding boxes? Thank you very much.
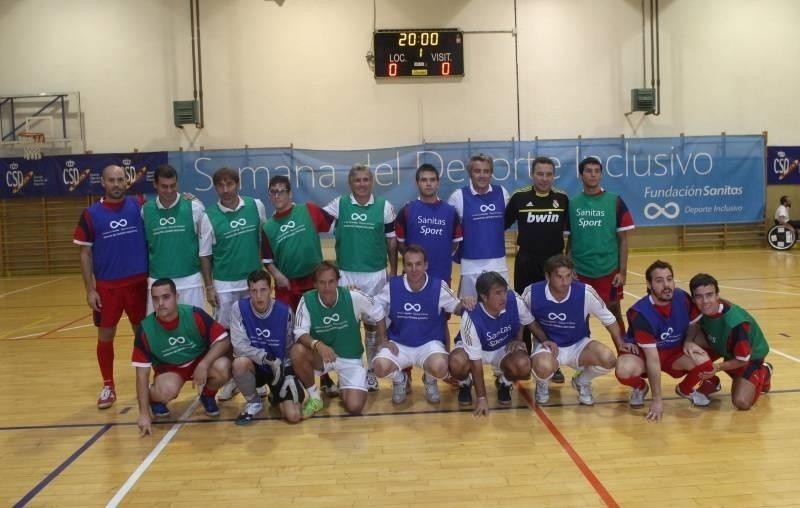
[615,260,772,423]
[774,195,800,232]
[73,154,634,440]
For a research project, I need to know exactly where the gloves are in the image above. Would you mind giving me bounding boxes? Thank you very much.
[264,354,283,386]
[279,375,310,405]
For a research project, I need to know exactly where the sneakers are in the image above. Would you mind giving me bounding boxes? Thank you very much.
[457,380,473,406]
[697,376,721,397]
[494,377,512,406]
[551,369,565,383]
[217,377,239,401]
[235,400,264,425]
[256,385,268,398]
[571,375,595,406]
[535,380,549,404]
[301,398,324,421]
[675,383,711,407]
[760,362,774,395]
[319,380,339,398]
[392,372,408,405]
[198,393,220,417]
[148,401,171,418]
[422,374,440,403]
[96,384,116,409]
[364,370,380,392]
[629,382,649,409]
